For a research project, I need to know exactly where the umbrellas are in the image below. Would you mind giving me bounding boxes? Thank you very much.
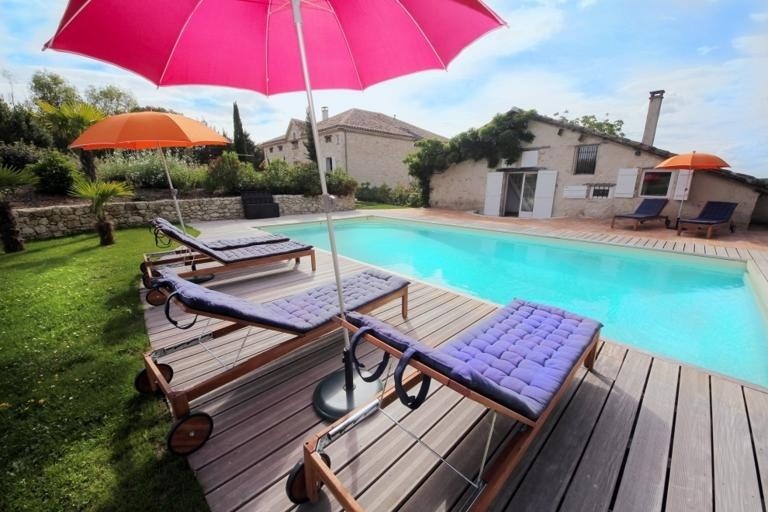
[653,150,731,229]
[38,0,511,389]
[68,110,231,250]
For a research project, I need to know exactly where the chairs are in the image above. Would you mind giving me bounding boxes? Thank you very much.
[677,201,738,239]
[135,269,411,456]
[143,224,316,306]
[286,298,604,512]
[140,217,289,273]
[611,199,671,231]
